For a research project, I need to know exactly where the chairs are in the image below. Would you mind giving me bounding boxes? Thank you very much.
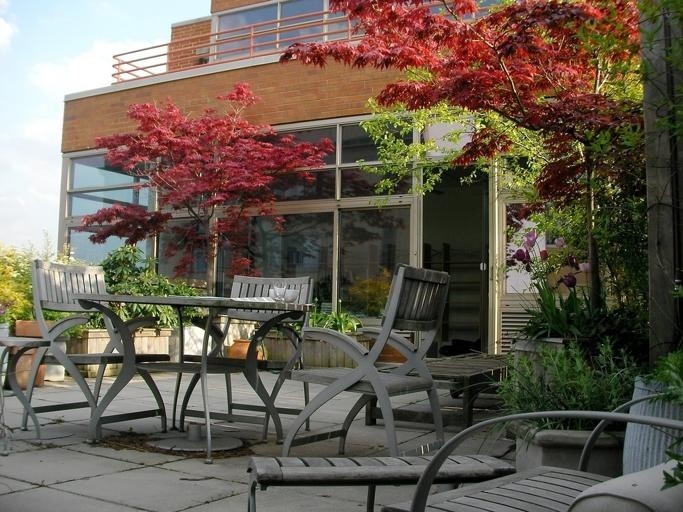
[279,263,454,457]
[379,381,682,512]
[166,274,314,440]
[25,261,172,441]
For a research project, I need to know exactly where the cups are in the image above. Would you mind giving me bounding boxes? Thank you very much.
[273,281,287,302]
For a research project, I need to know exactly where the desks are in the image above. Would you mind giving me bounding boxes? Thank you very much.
[73,292,313,455]
[1,337,58,456]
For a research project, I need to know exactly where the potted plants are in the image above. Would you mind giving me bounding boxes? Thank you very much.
[1,230,370,388]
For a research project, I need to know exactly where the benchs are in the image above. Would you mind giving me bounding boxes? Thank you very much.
[365,355,515,432]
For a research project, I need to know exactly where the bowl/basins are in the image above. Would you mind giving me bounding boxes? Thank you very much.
[269,288,300,303]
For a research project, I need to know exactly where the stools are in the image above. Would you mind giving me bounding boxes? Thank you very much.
[242,455,518,512]
